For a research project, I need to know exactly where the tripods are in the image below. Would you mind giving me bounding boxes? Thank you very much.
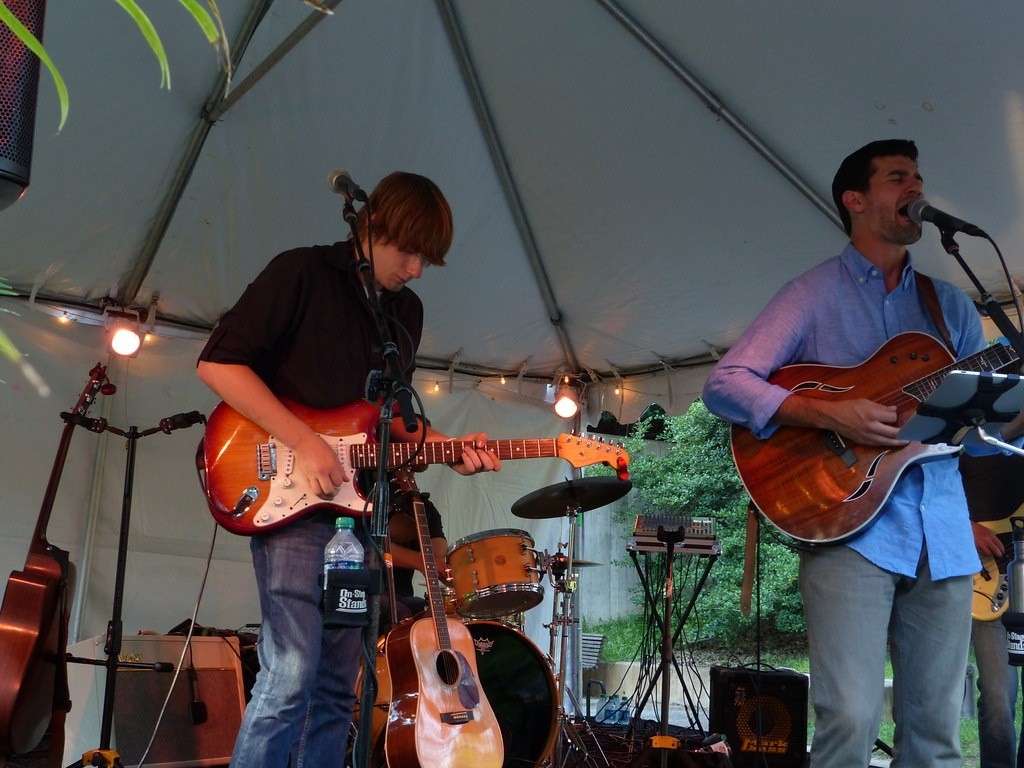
[545,501,616,768]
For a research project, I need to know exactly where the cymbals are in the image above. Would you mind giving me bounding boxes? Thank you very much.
[535,554,604,567]
[511,476,634,520]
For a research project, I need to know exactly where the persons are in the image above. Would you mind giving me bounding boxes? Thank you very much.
[363,463,446,652]
[701,139,1024,768]
[959,410,1024,768]
[196,170,500,768]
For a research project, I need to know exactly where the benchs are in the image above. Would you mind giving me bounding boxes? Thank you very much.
[582,633,606,698]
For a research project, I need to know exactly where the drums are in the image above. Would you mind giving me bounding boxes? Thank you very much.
[445,526,545,619]
[354,616,563,768]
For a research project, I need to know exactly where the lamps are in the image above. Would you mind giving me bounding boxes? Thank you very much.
[103,305,146,360]
[553,373,585,418]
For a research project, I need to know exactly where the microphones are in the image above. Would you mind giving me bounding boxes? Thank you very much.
[189,665,208,724]
[328,167,368,203]
[907,198,989,239]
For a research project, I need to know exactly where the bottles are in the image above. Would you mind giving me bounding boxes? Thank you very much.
[596,694,605,722]
[605,697,617,724]
[324,517,364,590]
[618,697,630,725]
[1007,516,1024,613]
[613,695,621,722]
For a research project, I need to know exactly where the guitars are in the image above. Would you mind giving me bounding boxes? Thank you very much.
[0,359,117,762]
[968,503,1024,624]
[729,330,1024,552]
[200,395,633,539]
[382,465,507,768]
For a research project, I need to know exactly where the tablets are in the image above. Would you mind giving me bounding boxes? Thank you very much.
[895,369,1024,445]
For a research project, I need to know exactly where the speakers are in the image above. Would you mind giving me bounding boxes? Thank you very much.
[64,633,246,768]
[708,665,809,753]
